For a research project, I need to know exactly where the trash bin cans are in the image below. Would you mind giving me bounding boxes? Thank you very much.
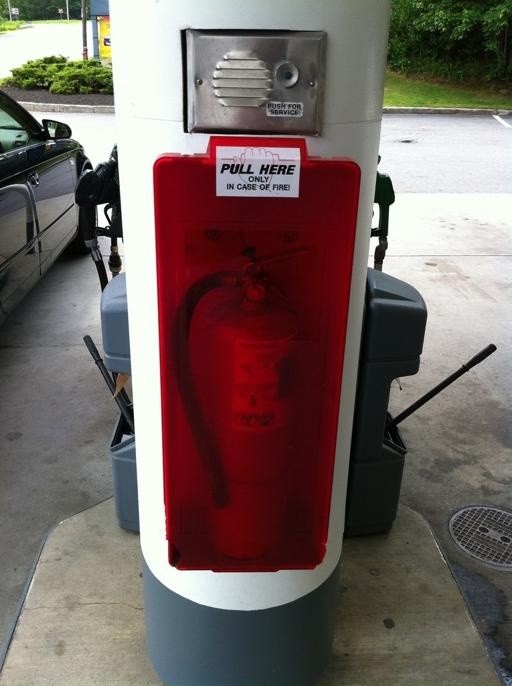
[344,269,429,536]
[101,273,138,546]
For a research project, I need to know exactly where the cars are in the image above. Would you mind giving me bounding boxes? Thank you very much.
[0,90,92,325]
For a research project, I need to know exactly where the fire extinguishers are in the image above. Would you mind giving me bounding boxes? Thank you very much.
[172,248,302,567]
